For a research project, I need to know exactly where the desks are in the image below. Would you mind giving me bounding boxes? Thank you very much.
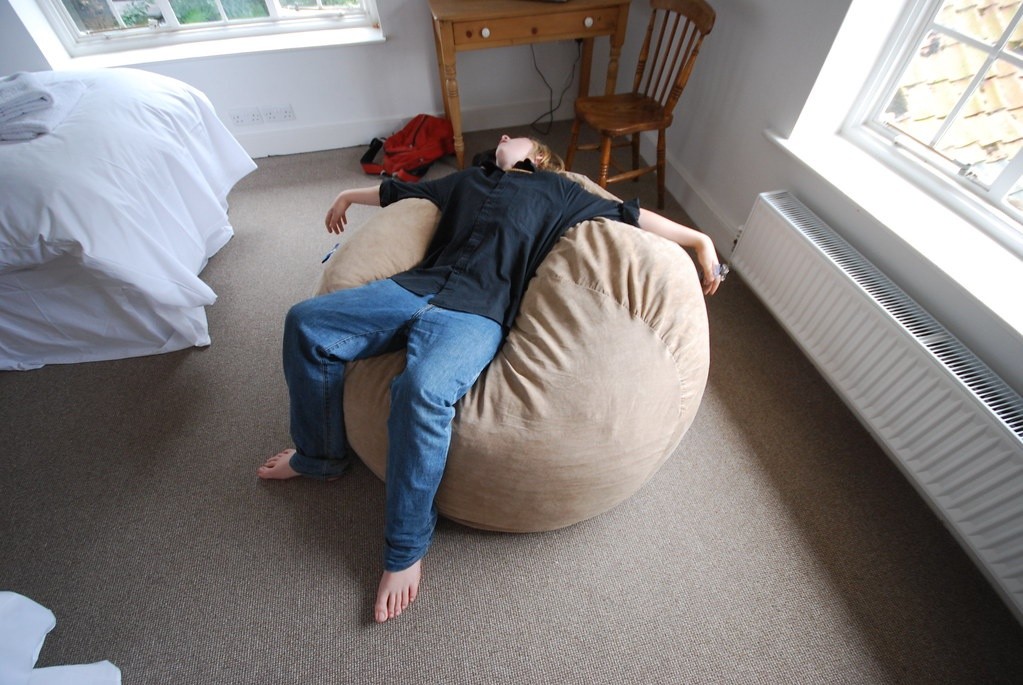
[427,0,631,173]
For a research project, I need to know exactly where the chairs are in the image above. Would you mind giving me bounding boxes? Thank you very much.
[564,0,717,213]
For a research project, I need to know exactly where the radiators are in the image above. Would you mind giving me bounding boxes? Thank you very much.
[726,191,1023,625]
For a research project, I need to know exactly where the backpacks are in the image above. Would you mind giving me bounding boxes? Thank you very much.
[360,114,456,183]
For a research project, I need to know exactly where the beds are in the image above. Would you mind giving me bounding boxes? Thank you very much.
[0,67,258,372]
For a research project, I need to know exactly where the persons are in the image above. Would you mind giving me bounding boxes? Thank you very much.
[257,134,721,623]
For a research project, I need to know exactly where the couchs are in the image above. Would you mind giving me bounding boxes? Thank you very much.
[313,197,710,534]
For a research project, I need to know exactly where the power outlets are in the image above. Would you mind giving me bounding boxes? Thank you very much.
[557,38,584,46]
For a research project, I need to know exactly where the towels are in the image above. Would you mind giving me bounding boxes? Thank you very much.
[0,70,92,140]
